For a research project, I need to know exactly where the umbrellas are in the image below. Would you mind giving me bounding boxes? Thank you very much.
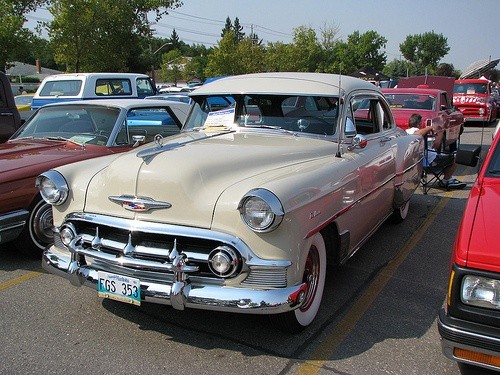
[458,58,500,79]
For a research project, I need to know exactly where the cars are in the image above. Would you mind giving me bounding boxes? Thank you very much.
[34,69,426,334]
[122,75,236,125]
[436,125,500,375]
[13,72,159,123]
[352,75,465,152]
[451,79,497,124]
[0,99,206,253]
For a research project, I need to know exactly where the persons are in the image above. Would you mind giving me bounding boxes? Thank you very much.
[19,86,27,95]
[490,81,500,109]
[405,113,467,188]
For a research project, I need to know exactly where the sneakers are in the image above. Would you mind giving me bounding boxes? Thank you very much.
[439,179,450,187]
[447,179,466,189]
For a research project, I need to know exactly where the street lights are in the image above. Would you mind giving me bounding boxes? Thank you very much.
[151,42,173,79]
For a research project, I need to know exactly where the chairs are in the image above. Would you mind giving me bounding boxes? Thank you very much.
[420,134,455,195]
